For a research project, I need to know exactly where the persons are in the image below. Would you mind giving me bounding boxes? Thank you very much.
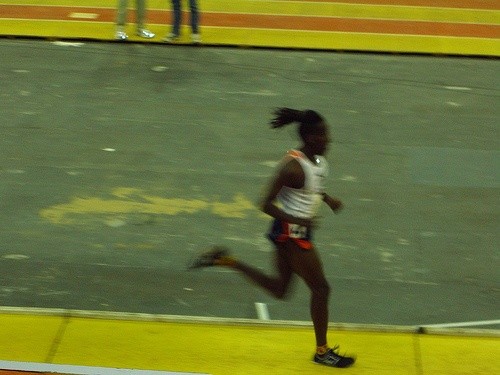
[116,0,156,40]
[184,106,357,369]
[167,0,203,38]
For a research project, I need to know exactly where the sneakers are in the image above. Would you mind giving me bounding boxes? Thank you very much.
[190,32,201,42]
[185,241,229,273]
[313,345,355,368]
[137,29,155,39]
[117,31,128,40]
[163,32,179,42]
